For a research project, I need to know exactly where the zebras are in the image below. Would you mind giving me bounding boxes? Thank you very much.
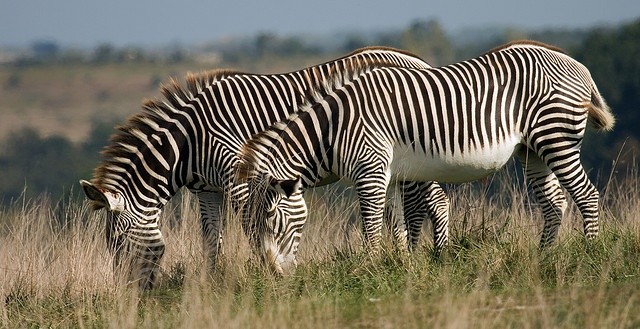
[232,38,614,278]
[78,45,453,293]
